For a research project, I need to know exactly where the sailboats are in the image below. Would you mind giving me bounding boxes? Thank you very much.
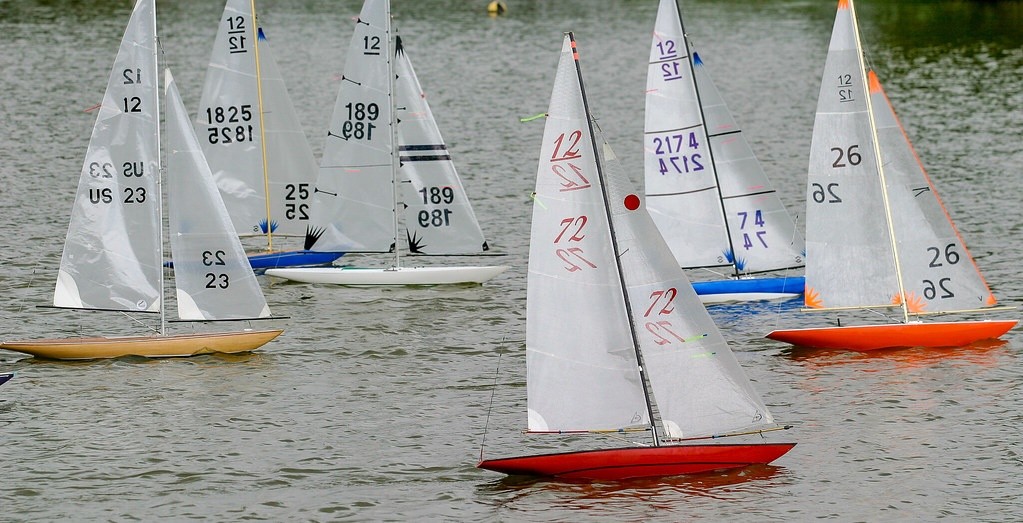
[765,1,1018,352]
[464,28,797,474]
[265,1,509,289]
[626,1,809,307]
[164,1,351,292]
[1,2,290,361]
